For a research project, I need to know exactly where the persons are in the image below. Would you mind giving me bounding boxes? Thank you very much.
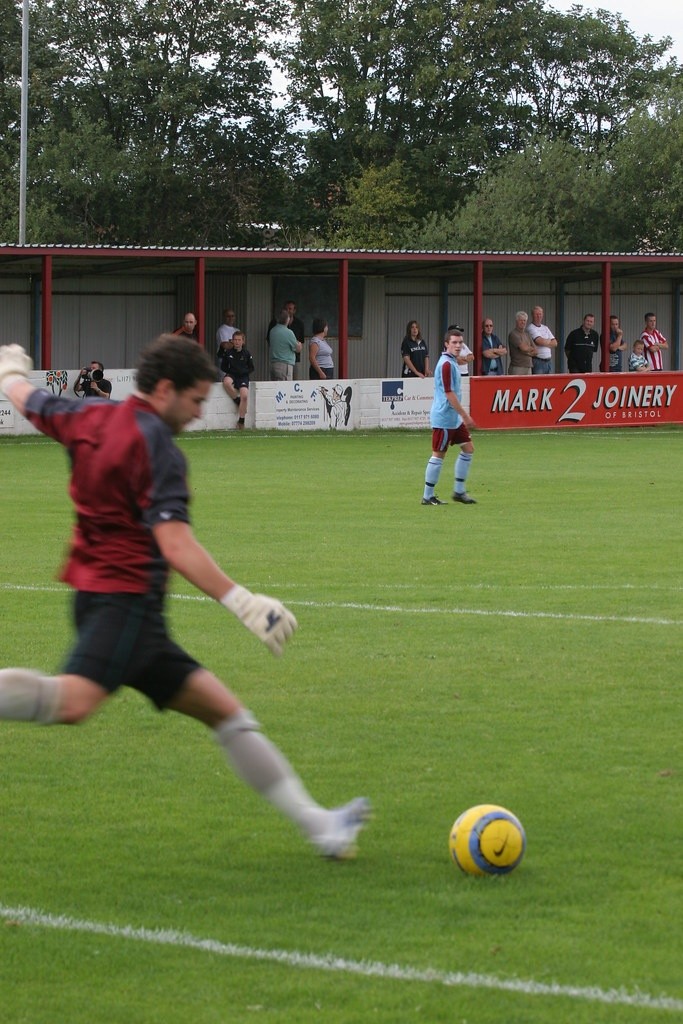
[639,312,668,371]
[628,340,651,373]
[267,301,305,381]
[564,313,599,373]
[599,315,626,372]
[171,307,255,432]
[482,319,507,376]
[421,331,477,506]
[73,361,112,400]
[401,320,433,379]
[309,319,334,380]
[0,334,370,861]
[508,311,539,375]
[444,325,474,376]
[528,306,557,374]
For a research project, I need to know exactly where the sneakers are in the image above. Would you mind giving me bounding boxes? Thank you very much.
[451,491,477,504]
[421,496,448,505]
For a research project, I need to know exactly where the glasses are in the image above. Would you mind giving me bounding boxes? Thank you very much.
[485,324,493,327]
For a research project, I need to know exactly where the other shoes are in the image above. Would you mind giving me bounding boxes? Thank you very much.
[236,421,246,431]
[307,795,371,856]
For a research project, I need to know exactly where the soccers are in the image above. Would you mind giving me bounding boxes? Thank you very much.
[449,804,526,880]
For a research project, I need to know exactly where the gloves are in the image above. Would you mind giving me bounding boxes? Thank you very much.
[1,341,34,378]
[241,591,300,658]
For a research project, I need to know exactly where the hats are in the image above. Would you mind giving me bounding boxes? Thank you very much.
[332,384,344,396]
[448,325,465,332]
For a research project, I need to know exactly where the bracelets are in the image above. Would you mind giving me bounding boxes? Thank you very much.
[97,389,100,393]
